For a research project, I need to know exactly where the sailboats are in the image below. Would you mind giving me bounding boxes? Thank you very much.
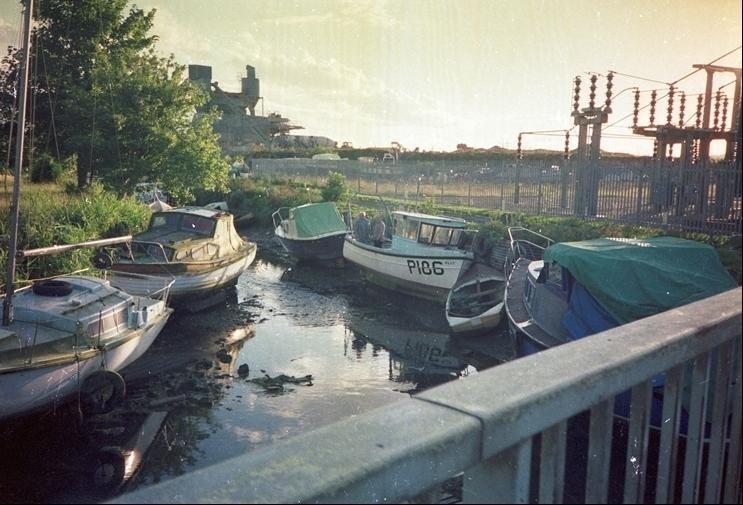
[0,0,175,426]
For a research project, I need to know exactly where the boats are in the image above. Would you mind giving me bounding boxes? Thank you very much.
[504,226,736,452]
[271,203,516,338]
[105,200,257,300]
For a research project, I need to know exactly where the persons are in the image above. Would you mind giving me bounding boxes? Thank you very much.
[371,215,386,247]
[353,210,372,247]
